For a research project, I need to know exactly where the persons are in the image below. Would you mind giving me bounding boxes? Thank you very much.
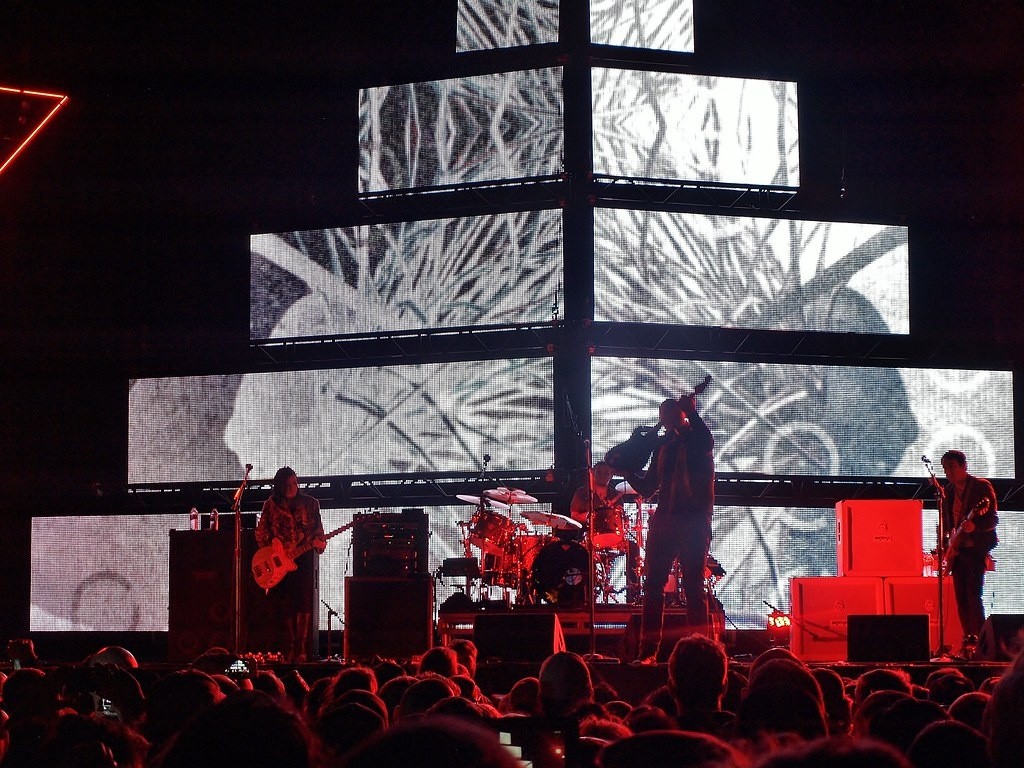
[254,466,327,663]
[931,450,999,661]
[0,633,1024,767]
[570,461,645,604]
[610,394,714,666]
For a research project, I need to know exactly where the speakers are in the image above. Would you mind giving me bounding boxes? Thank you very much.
[474,613,568,662]
[167,528,319,661]
[788,500,1024,663]
[342,576,433,664]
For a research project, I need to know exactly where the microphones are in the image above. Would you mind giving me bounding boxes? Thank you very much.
[922,455,932,463]
[245,464,253,470]
[484,455,491,466]
[812,635,819,641]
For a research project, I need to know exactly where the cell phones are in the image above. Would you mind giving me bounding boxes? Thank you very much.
[224,659,251,675]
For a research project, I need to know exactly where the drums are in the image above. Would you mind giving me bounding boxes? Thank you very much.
[586,506,624,548]
[513,533,560,587]
[482,535,521,589]
[529,540,605,605]
[468,510,518,557]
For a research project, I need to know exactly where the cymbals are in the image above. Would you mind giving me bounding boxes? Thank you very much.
[483,487,539,505]
[615,481,638,495]
[456,494,511,509]
[519,509,582,530]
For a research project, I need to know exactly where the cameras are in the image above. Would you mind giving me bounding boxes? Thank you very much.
[100,698,117,717]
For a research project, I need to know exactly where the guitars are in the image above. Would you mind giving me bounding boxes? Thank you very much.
[603,375,712,481]
[938,497,990,576]
[251,512,382,591]
